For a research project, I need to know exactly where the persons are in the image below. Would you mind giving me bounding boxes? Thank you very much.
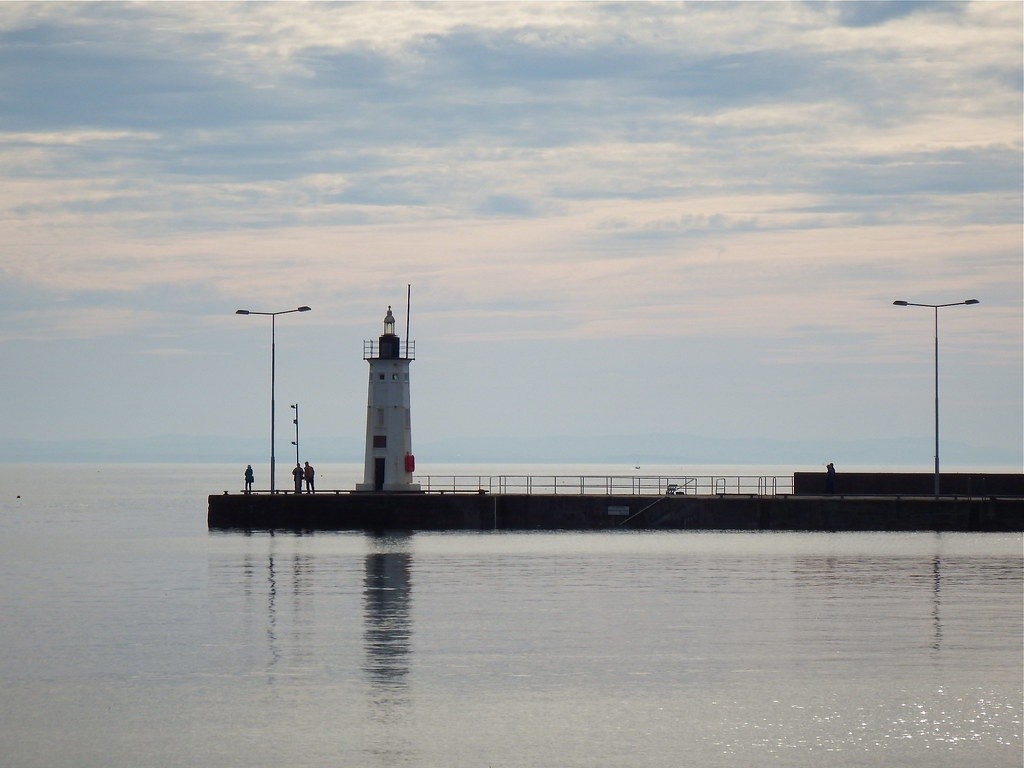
[826,463,835,473]
[292,463,304,494]
[245,465,253,494]
[304,461,315,494]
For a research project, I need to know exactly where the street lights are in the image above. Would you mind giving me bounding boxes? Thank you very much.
[893,299,981,493]
[235,305,313,494]
